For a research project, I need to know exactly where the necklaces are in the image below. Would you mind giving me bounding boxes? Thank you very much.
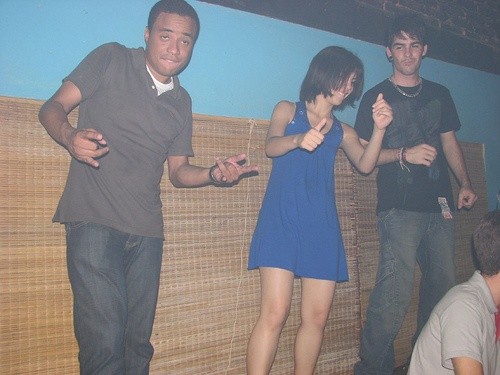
[389,75,423,97]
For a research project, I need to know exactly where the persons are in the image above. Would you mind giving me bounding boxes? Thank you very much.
[354,14,478,375]
[246,45,393,375]
[38,0,259,375]
[407,210,500,375]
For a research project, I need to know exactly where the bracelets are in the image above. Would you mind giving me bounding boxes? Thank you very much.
[399,147,411,172]
[209,165,221,186]
